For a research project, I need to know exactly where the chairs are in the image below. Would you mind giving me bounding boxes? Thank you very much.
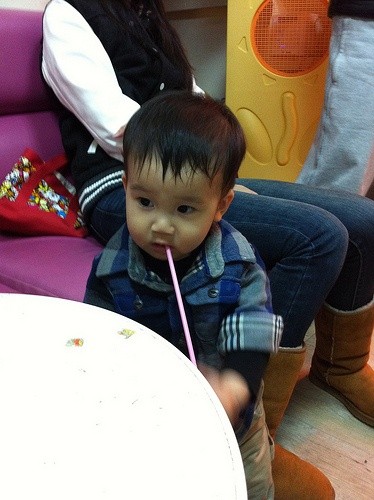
[0,0,104,302]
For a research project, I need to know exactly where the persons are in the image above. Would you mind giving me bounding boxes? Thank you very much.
[38,0,374,499]
[83,89,284,499]
[292,1,373,196]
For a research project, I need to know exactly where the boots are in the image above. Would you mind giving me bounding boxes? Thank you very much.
[263,341,335,500]
[309,297,373,430]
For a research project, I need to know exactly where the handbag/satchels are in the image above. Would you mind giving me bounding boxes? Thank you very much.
[0,147,86,238]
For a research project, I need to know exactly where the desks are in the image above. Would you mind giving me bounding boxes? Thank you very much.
[0,293,248,500]
